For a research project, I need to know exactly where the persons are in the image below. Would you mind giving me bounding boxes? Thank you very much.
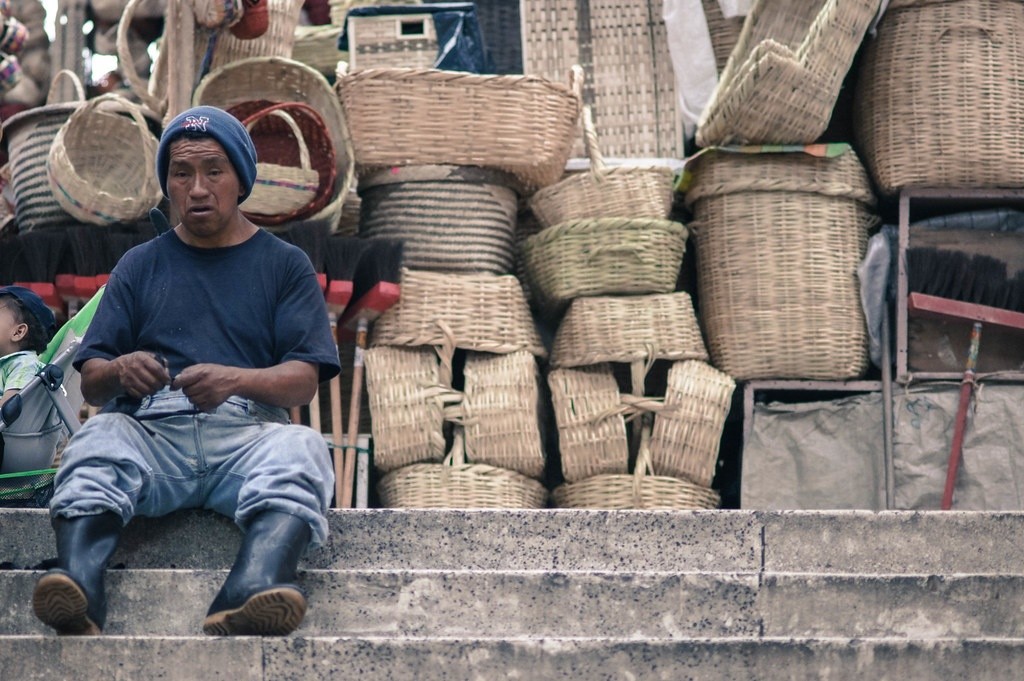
[34,106,343,634]
[0,286,56,437]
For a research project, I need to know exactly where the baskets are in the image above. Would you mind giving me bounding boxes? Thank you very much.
[0,0,1024,511]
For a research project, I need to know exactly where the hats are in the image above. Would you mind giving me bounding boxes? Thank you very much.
[0,286,56,343]
[157,104,257,206]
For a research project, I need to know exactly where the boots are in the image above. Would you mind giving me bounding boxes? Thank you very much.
[203,511,312,636]
[34,510,122,638]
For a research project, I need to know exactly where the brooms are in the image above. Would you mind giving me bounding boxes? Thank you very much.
[905,246,1024,510]
[289,214,406,509]
[3,219,159,330]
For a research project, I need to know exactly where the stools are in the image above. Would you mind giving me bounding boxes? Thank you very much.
[324,432,373,509]
